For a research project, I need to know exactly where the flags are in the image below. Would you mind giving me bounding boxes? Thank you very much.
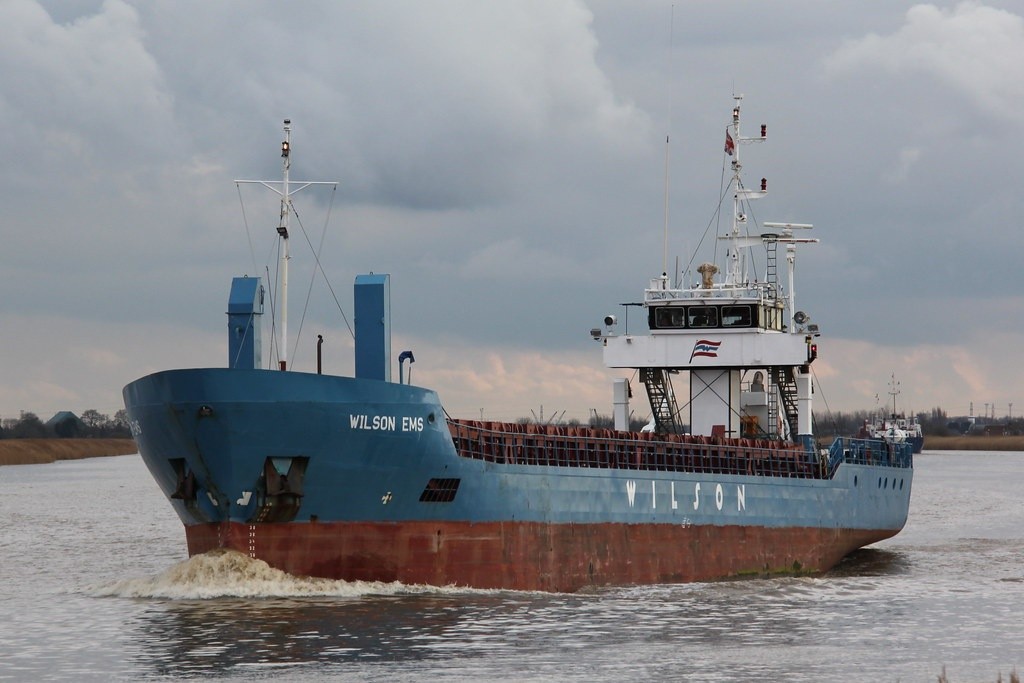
[723,128,734,156]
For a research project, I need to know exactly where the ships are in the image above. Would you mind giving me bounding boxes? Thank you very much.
[119,88,927,582]
